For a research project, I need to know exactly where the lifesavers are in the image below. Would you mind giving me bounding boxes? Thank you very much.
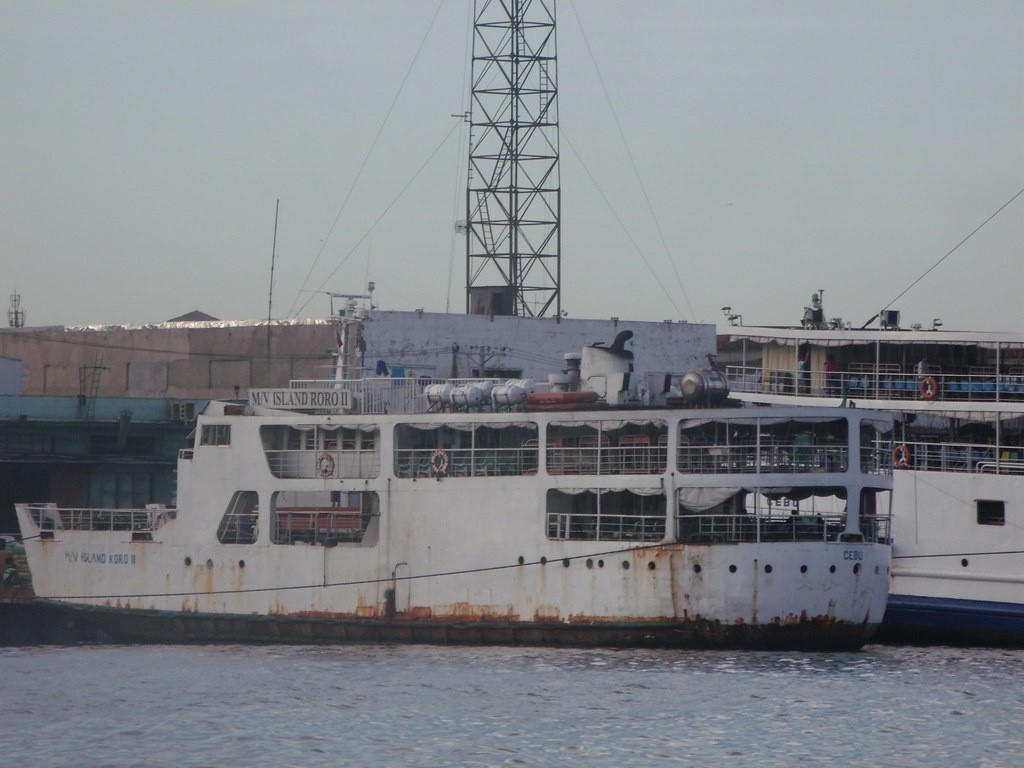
[920,376,939,400]
[431,450,449,473]
[892,446,912,468]
[316,452,334,477]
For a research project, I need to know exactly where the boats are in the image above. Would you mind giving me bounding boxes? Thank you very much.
[718,320,1024,642]
[10,292,903,649]
